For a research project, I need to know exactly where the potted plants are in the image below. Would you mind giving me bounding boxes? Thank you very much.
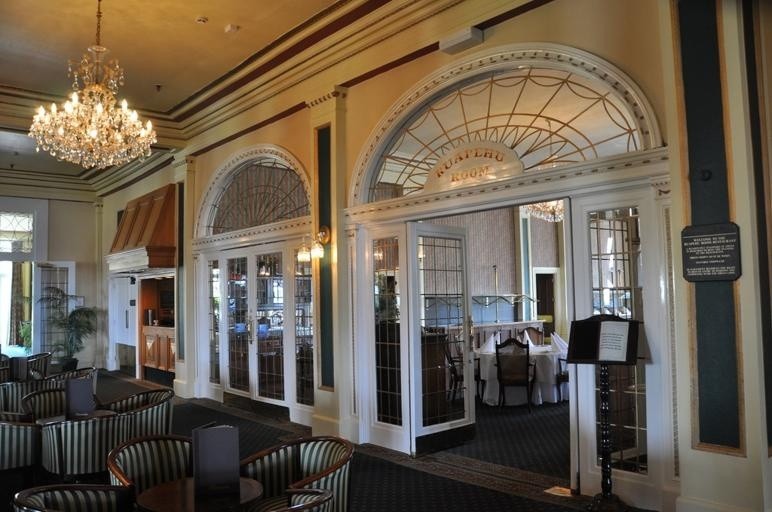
[33,281,110,372]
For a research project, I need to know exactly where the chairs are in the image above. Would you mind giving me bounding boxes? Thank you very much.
[443,326,572,417]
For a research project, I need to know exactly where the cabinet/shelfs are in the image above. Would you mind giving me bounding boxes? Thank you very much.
[376,331,451,430]
[430,319,548,403]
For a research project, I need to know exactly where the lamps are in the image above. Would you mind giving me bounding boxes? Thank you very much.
[295,227,331,264]
[524,115,568,225]
[28,2,160,171]
